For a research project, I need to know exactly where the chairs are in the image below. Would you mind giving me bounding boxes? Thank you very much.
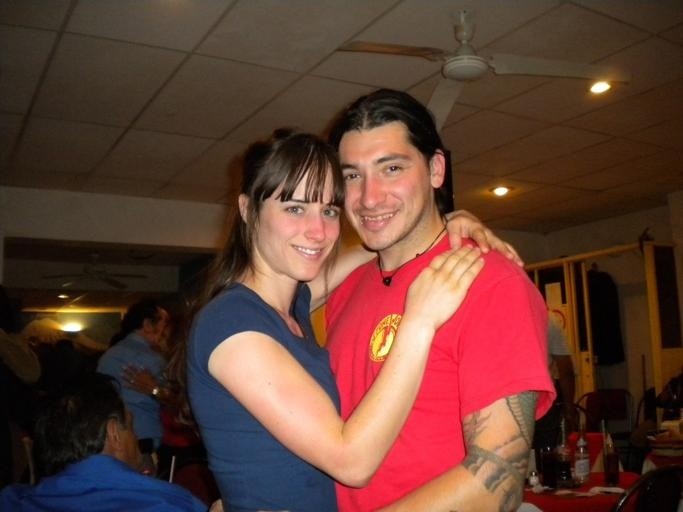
[517,374,682,512]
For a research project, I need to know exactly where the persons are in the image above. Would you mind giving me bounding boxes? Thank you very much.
[318,89,557,511]
[532,308,574,488]
[629,371,683,450]
[184,123,524,512]
[0,298,224,512]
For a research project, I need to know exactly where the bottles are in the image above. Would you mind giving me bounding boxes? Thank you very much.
[528,471,538,486]
[553,416,590,485]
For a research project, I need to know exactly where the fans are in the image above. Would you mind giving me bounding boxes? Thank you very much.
[42,262,146,287]
[339,7,630,135]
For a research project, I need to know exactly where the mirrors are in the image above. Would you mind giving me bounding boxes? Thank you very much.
[1,237,227,364]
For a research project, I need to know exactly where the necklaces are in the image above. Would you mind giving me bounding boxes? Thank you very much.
[370,224,448,286]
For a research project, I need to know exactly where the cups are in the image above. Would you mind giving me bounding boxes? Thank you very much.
[539,444,557,489]
[601,450,622,485]
